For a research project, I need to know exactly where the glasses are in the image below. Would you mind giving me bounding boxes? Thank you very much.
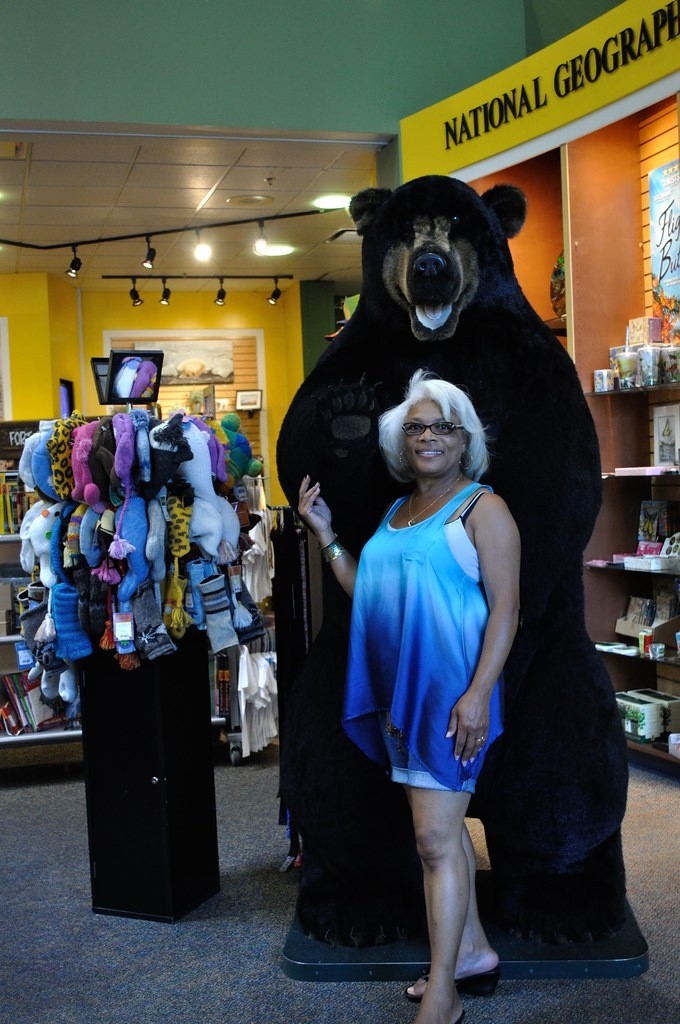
[401,422,464,436]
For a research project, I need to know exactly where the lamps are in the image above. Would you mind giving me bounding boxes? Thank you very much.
[0,194,358,308]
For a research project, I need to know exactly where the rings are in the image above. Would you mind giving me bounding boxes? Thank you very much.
[476,735,486,744]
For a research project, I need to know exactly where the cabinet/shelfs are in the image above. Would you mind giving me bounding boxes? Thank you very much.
[0,419,263,772]
[576,384,679,778]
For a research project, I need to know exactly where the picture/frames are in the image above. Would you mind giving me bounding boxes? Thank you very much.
[133,340,234,386]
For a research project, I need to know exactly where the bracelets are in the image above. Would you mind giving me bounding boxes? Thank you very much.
[317,533,347,566]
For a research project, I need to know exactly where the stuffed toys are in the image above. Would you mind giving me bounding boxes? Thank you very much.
[276,172,632,954]
[19,354,265,721]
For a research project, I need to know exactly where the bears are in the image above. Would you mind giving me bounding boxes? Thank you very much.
[275,176,630,950]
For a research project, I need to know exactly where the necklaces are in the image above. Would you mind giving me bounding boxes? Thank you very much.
[407,473,464,527]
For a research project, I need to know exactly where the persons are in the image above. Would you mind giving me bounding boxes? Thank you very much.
[295,366,523,1024]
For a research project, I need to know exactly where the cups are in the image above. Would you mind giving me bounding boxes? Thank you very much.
[661,347,680,384]
[654,641,666,658]
[648,644,658,660]
[616,352,638,390]
[592,368,615,394]
[637,346,660,387]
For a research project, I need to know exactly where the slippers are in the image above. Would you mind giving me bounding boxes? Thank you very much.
[404,965,502,1002]
